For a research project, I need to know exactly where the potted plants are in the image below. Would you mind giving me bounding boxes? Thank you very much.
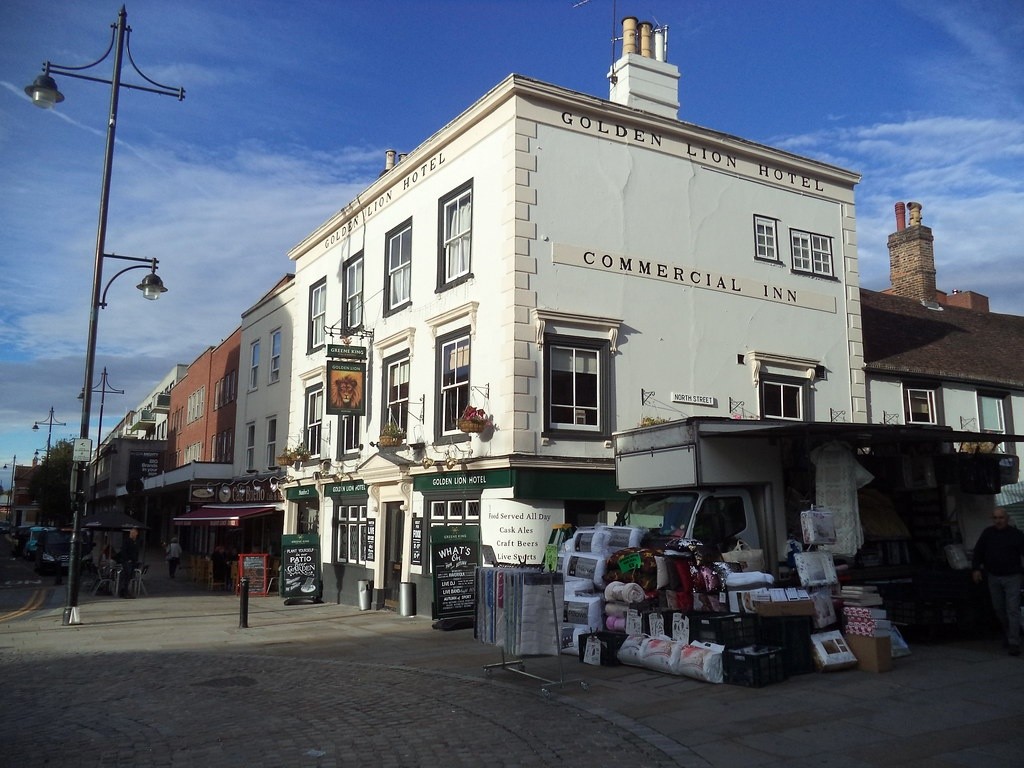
[290,442,312,463]
[277,446,295,467]
[379,418,407,447]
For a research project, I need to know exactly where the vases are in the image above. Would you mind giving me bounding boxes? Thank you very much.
[458,418,485,432]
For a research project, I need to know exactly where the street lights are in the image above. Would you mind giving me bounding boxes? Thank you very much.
[31,405,66,525]
[76,365,125,552]
[3,454,16,523]
[23,5,188,624]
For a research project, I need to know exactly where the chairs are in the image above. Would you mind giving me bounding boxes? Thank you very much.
[87,557,152,600]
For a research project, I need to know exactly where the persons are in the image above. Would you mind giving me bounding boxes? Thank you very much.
[119,528,138,599]
[971,506,1024,656]
[165,537,182,578]
[211,546,232,591]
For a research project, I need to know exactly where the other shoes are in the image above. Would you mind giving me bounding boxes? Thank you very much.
[119,593,135,599]
[1003,641,1023,655]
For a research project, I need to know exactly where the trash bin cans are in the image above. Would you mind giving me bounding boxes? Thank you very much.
[398,582,415,617]
[357,579,373,610]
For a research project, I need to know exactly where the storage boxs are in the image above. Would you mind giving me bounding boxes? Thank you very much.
[846,630,893,675]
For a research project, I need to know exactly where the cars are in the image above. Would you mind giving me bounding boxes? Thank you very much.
[35,528,96,572]
[25,527,56,557]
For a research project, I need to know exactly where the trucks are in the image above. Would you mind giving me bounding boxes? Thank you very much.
[613,411,1024,587]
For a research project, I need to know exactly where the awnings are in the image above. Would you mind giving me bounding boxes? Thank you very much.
[172,503,276,530]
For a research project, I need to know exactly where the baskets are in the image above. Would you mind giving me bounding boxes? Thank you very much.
[578,632,627,666]
[722,644,788,688]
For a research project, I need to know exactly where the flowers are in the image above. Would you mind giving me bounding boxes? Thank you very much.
[459,406,500,442]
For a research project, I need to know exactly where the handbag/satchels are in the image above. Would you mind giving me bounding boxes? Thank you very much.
[165,554,172,561]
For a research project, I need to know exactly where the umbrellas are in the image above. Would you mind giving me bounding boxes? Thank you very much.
[78,511,150,593]
